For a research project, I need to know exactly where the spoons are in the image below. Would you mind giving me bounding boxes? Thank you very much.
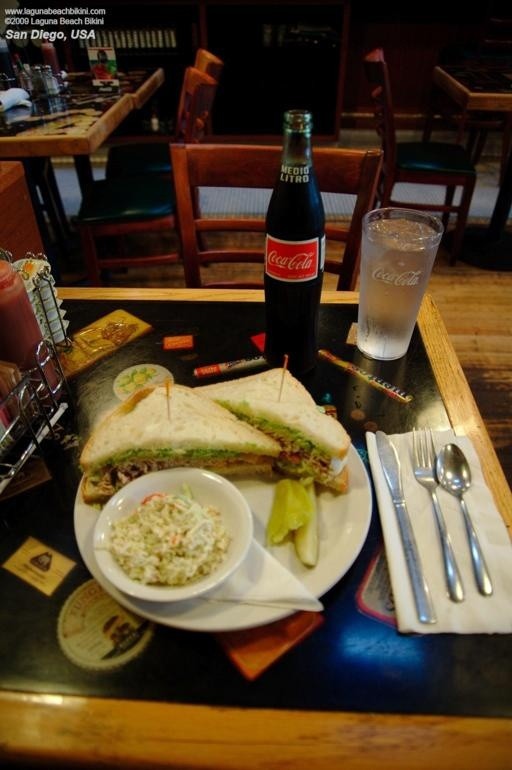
[435,442,496,599]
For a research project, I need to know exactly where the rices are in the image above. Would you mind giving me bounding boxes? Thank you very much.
[109,491,231,587]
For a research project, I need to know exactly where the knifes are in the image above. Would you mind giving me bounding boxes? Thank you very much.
[373,429,438,623]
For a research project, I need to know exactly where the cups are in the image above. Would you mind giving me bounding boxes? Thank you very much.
[357,207,444,362]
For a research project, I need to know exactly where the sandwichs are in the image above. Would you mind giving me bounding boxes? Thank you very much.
[79,380,282,506]
[196,367,353,496]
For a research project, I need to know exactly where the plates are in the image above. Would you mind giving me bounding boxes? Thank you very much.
[73,448,373,634]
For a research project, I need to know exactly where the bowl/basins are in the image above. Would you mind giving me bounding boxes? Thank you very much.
[93,465,254,604]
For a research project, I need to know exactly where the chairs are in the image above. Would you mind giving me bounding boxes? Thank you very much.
[2,48,511,290]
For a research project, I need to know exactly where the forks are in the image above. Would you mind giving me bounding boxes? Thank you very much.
[409,425,466,604]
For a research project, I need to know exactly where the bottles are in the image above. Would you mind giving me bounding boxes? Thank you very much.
[12,54,60,98]
[259,108,330,375]
[0,260,62,403]
